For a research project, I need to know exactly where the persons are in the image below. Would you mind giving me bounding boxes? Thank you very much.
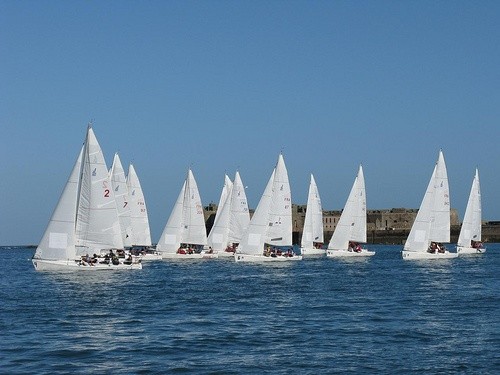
[178,245,194,255]
[349,242,362,252]
[81,246,146,266]
[265,245,295,257]
[430,242,445,253]
[208,246,212,254]
[471,241,483,248]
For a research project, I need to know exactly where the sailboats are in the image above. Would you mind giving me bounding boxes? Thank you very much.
[31,122,143,273]
[456,168,486,254]
[326,164,375,257]
[153,168,208,259]
[300,173,326,255]
[401,148,459,261]
[108,152,163,261]
[233,149,303,262]
[199,170,251,257]
